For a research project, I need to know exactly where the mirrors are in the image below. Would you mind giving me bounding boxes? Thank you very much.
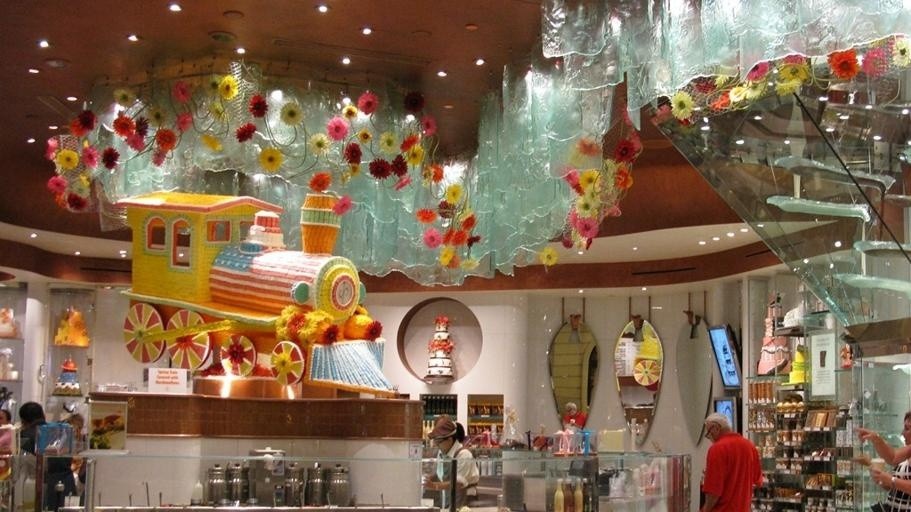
[546,319,601,429]
[613,316,664,446]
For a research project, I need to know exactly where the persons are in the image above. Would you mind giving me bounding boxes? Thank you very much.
[0,409,11,477]
[15,401,78,512]
[701,413,763,512]
[421,417,480,509]
[854,412,911,512]
[563,402,587,430]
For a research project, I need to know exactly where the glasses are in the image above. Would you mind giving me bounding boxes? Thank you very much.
[705,425,714,439]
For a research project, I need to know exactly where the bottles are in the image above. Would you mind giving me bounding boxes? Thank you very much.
[562,479,573,512]
[573,477,583,511]
[554,479,563,512]
[583,478,592,512]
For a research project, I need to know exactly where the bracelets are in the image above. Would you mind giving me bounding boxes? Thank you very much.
[891,476,896,489]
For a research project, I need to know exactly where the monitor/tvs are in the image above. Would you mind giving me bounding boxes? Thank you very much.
[709,323,742,389]
[713,397,742,435]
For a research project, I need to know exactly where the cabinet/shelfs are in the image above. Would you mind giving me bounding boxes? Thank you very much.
[466,394,506,444]
[0,279,29,512]
[746,271,911,512]
[47,289,95,509]
[83,387,439,512]
[423,445,692,512]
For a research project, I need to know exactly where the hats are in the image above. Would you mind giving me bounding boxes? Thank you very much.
[427,418,457,439]
